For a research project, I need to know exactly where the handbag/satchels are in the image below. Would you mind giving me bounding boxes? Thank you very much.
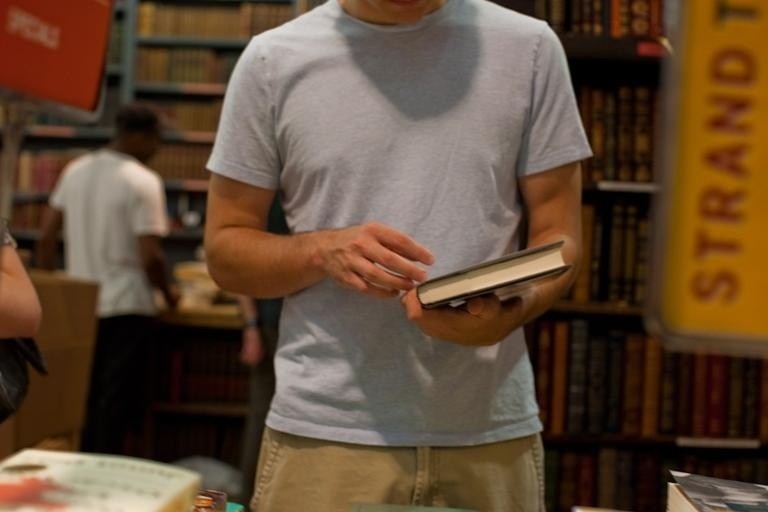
[0,337,48,424]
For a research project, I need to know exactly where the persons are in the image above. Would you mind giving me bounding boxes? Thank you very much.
[36,104,179,455]
[204,1,595,512]
[1,210,50,426]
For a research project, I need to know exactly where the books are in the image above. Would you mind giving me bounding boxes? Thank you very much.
[505,1,768,512]
[4,16,126,239]
[140,4,291,225]
[160,257,245,459]
[415,236,568,313]
